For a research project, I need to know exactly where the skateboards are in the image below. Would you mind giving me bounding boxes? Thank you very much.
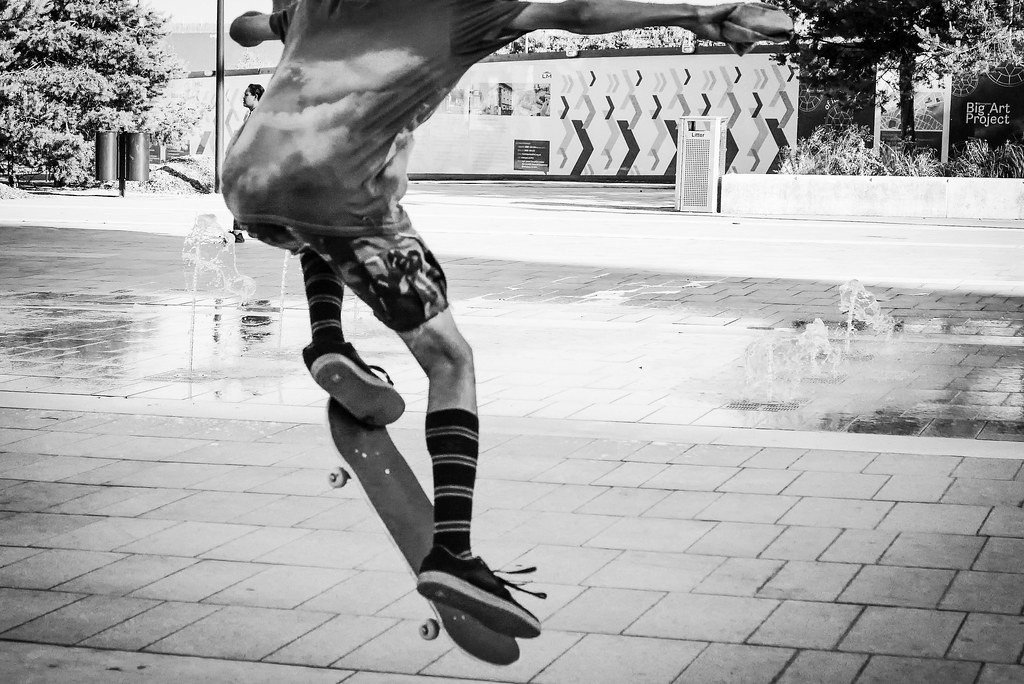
[325,393,521,666]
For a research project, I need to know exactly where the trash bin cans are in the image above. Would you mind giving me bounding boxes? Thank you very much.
[673,116,730,214]
[95,129,124,180]
[126,132,149,181]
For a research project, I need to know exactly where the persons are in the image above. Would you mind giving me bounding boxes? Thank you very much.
[218,83,264,245]
[217,0,749,642]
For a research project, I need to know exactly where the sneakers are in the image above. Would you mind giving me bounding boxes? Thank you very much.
[302,340,405,424]
[415,550,547,640]
[217,231,245,244]
